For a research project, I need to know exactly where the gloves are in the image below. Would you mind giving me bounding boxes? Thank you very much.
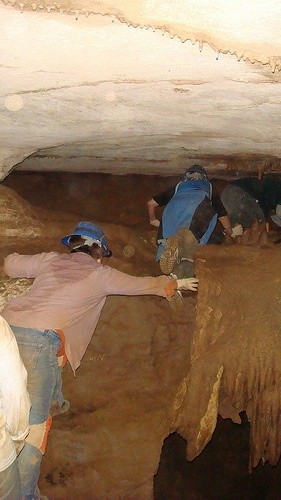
[175,278,198,291]
[231,225,242,237]
[150,219,160,227]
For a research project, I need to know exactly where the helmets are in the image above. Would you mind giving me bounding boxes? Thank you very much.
[61,221,112,257]
[184,165,207,176]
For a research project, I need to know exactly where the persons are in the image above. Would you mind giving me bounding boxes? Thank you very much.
[147,165,232,305]
[0,221,199,500]
[0,315,31,500]
[222,174,281,244]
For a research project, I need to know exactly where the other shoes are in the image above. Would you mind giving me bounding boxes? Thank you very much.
[160,236,178,274]
[50,399,70,416]
[165,273,183,301]
